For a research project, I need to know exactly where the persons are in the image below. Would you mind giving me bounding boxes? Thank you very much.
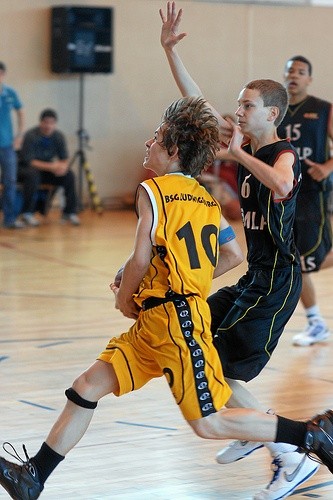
[159,1,320,500]
[0,62,26,230]
[276,55,333,346]
[18,108,82,226]
[0,97,333,500]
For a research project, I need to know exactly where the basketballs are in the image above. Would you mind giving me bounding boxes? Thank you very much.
[226,199,242,219]
[115,264,140,310]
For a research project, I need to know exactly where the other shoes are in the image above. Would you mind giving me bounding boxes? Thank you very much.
[9,219,24,228]
[68,214,80,225]
[23,213,40,226]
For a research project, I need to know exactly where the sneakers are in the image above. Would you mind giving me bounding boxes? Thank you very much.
[253,448,320,500]
[215,409,275,464]
[295,409,333,474]
[0,442,44,500]
[293,322,331,346]
